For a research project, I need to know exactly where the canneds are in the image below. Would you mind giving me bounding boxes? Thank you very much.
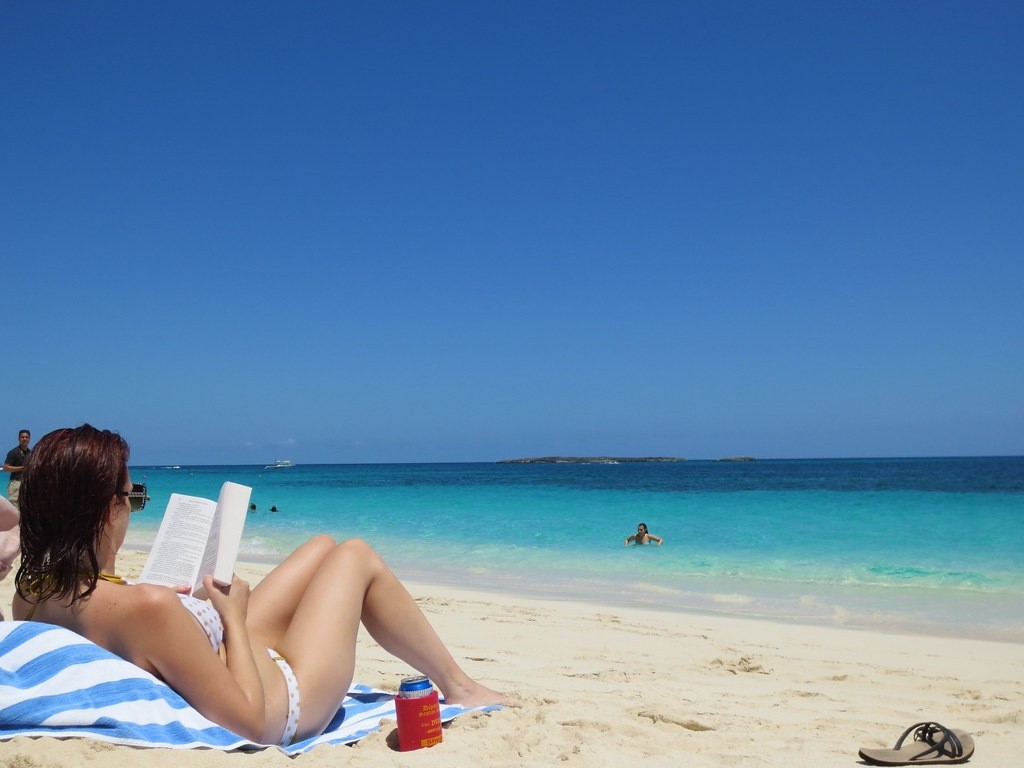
[398,675,433,699]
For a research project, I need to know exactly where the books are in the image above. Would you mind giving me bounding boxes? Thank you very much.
[137,481,253,600]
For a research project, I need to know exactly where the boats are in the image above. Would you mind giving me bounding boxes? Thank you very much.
[265,461,297,467]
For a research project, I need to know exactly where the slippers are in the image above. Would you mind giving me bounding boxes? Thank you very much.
[859,722,975,766]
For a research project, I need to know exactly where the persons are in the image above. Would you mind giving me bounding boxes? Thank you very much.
[12,423,526,746]
[625,523,663,544]
[0,430,32,581]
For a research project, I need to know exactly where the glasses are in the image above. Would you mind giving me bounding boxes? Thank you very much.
[113,484,151,513]
